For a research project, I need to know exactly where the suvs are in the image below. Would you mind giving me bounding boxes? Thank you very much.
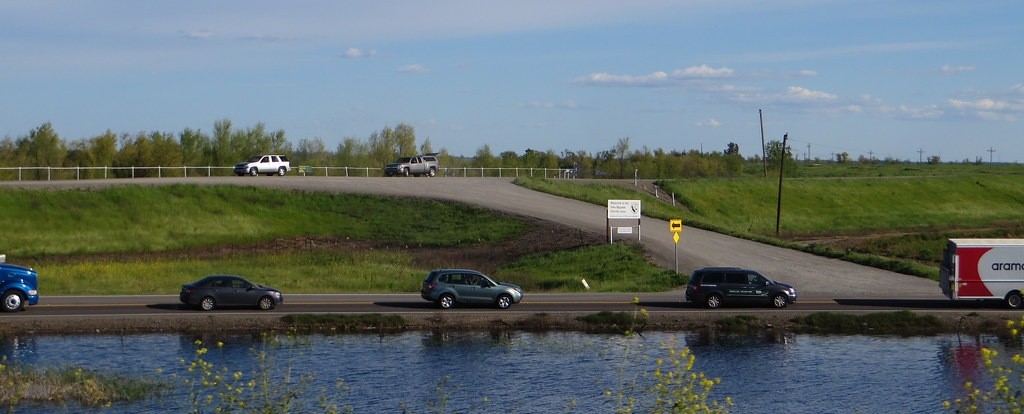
[422,269,523,309]
[686,267,798,308]
[233,154,291,176]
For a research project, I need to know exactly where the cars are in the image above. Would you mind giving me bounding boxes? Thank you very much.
[180,275,283,310]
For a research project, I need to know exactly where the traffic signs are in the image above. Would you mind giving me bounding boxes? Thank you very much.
[670,219,682,232]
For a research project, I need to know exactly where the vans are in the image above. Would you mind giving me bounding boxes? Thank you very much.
[0,263,39,313]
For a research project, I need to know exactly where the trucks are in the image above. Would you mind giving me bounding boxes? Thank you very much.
[939,238,1024,308]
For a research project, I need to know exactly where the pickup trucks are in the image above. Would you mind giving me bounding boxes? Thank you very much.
[384,153,439,177]
